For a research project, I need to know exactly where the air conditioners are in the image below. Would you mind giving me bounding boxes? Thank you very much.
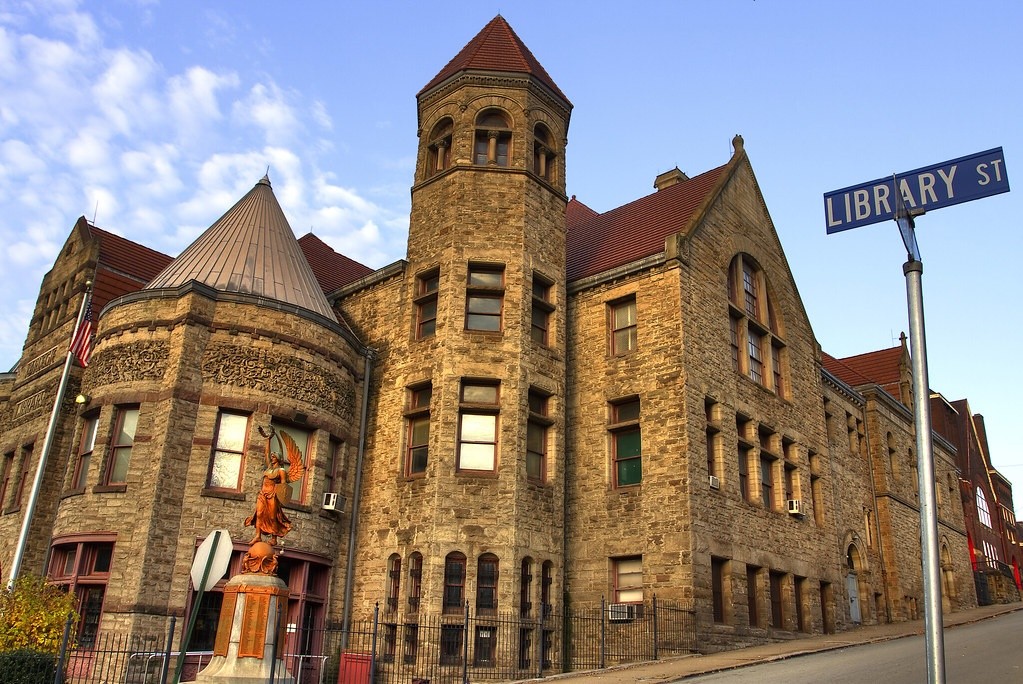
[709,474,721,489]
[321,492,349,516]
[786,498,808,516]
[606,603,639,621]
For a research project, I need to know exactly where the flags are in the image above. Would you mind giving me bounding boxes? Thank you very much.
[68,288,93,368]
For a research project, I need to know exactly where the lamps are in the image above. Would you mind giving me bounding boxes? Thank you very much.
[75,392,92,405]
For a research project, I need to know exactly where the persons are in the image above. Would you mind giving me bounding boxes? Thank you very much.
[244,433,294,546]
